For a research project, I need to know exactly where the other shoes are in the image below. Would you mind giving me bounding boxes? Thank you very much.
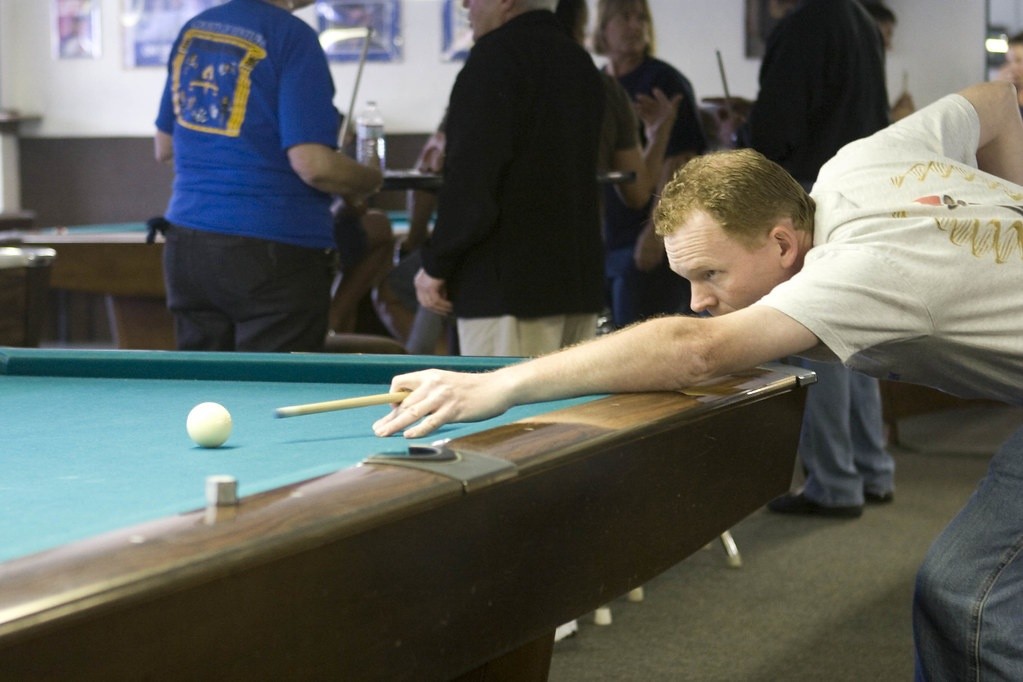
[769,490,862,518]
[862,487,896,504]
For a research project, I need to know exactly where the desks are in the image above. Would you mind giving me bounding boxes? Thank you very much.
[0,209,818,682]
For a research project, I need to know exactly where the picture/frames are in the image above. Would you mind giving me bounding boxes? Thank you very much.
[315,0,401,62]
[50,0,102,60]
[125,0,230,69]
[441,0,474,63]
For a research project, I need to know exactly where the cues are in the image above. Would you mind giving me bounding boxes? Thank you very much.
[274,391,408,421]
[901,68,910,95]
[335,24,376,154]
[715,50,740,129]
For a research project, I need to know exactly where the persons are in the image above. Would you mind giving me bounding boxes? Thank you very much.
[701,0,898,519]
[998,33,1023,119]
[154,0,382,354]
[371,80,1023,682]
[372,1,711,355]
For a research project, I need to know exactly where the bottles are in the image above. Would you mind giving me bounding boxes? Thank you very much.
[355,100,385,172]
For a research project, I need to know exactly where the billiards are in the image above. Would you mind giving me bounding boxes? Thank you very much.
[185,400,233,446]
